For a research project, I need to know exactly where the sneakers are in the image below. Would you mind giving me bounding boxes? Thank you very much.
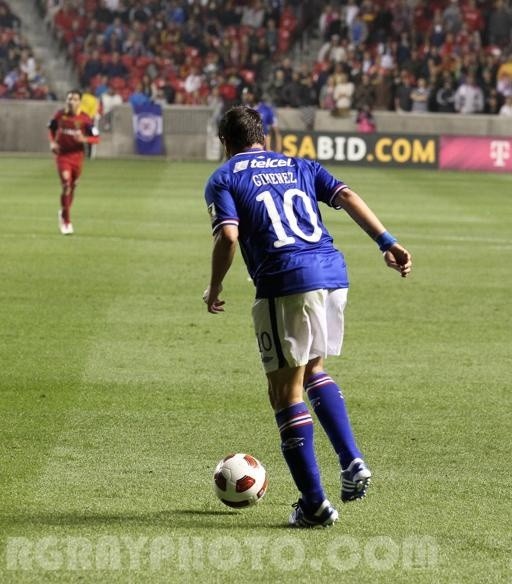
[338,455,372,504]
[56,210,76,237]
[287,497,342,529]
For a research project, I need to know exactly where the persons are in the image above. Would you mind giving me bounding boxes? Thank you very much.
[201,104,414,533]
[1,0,512,158]
[45,90,102,236]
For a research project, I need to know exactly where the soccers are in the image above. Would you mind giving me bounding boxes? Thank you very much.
[213,453,268,509]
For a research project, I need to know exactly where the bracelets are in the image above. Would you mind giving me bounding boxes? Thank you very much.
[376,231,399,254]
